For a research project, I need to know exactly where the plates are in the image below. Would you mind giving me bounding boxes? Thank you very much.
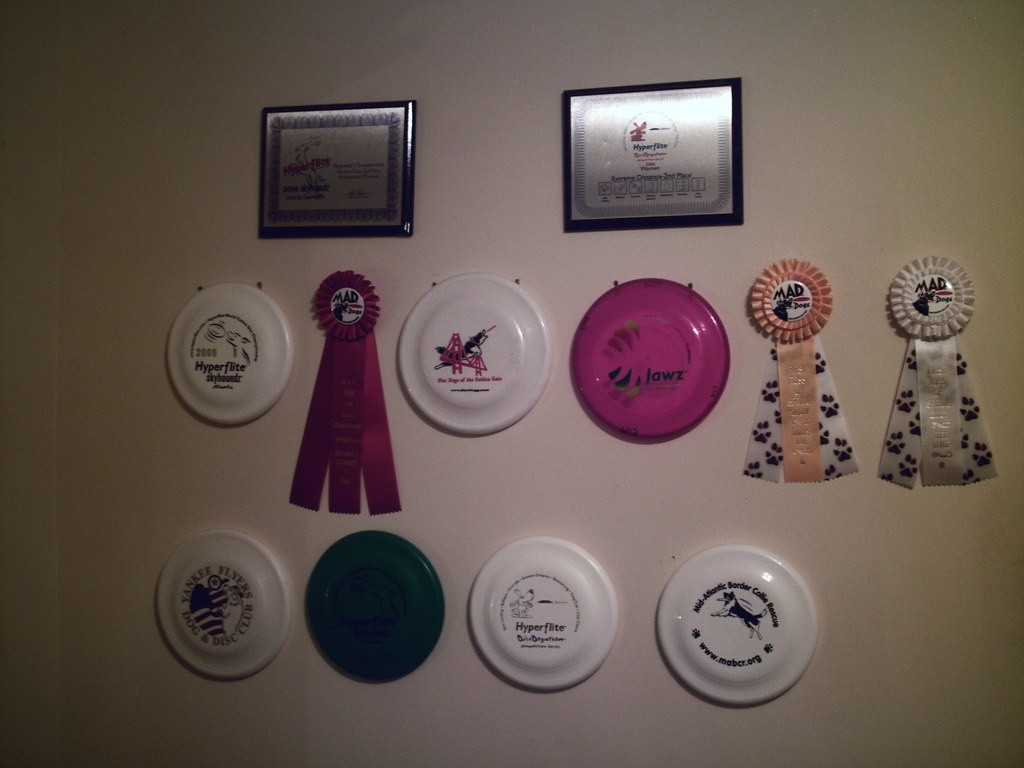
[570,278,730,441]
[467,534,621,694]
[157,527,293,680]
[301,531,444,683]
[397,271,552,439]
[163,283,294,428]
[657,544,819,708]
